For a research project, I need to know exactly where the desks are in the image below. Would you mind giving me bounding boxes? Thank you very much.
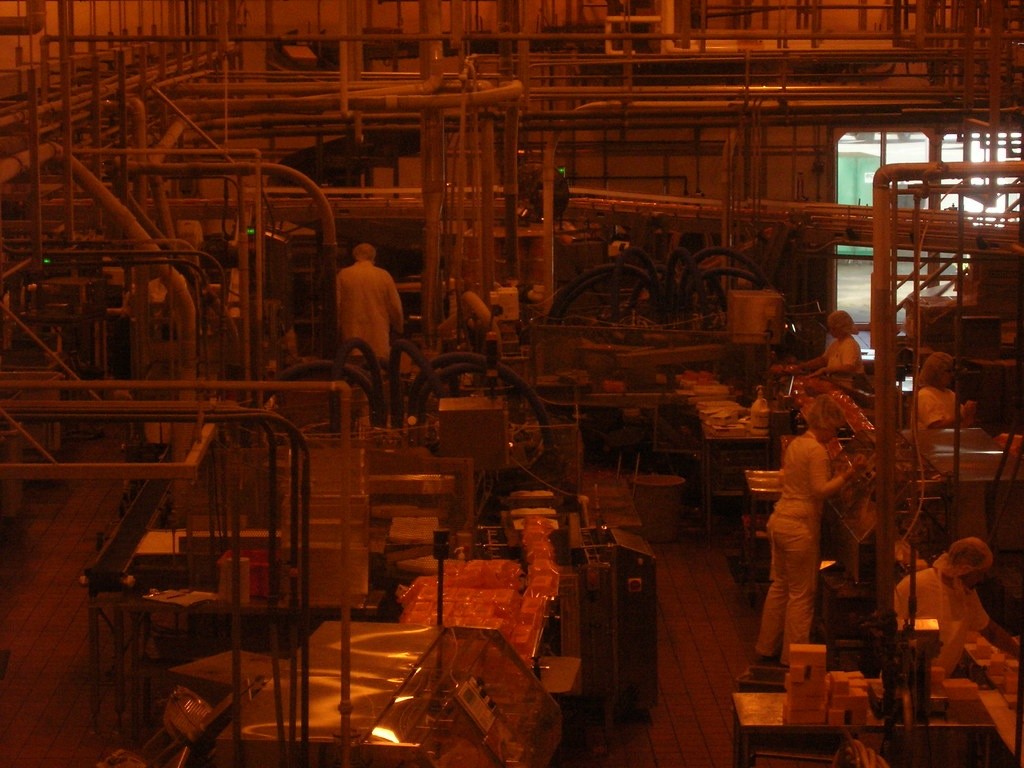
[741,470,860,607]
[733,687,996,768]
[701,399,1024,535]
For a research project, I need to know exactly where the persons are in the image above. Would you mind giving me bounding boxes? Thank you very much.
[336,244,403,429]
[755,310,1020,678]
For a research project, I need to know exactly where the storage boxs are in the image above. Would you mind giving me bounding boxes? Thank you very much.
[438,396,507,465]
[783,638,1020,726]
[906,255,1024,426]
[727,289,782,343]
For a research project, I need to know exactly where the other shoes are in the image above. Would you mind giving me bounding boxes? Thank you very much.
[749,646,781,663]
